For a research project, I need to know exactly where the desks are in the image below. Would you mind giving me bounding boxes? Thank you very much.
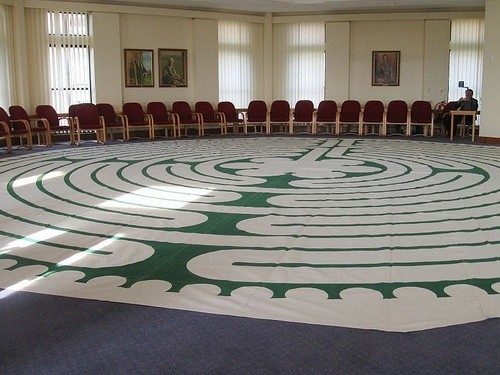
[450,110,480,142]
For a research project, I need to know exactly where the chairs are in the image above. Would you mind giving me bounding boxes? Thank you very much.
[0,100,477,153]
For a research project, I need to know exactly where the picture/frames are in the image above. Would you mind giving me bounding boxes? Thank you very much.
[372,51,400,86]
[158,48,188,87]
[124,48,154,88]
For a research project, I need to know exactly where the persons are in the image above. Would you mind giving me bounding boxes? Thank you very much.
[443,89,478,140]
